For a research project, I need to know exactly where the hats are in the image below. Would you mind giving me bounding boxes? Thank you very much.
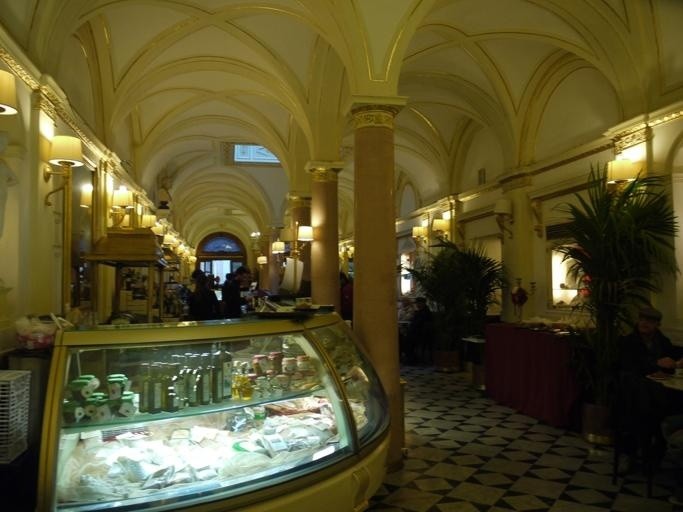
[638,307,662,322]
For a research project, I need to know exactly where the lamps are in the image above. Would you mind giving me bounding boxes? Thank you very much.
[42,135,85,207]
[107,189,133,228]
[0,69,18,117]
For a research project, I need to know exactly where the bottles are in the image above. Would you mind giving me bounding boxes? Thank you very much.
[137,341,233,416]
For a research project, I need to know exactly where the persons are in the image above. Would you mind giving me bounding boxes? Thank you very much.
[339,271,435,366]
[178,265,255,319]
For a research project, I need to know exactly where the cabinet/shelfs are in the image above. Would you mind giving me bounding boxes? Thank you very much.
[35,307,393,511]
